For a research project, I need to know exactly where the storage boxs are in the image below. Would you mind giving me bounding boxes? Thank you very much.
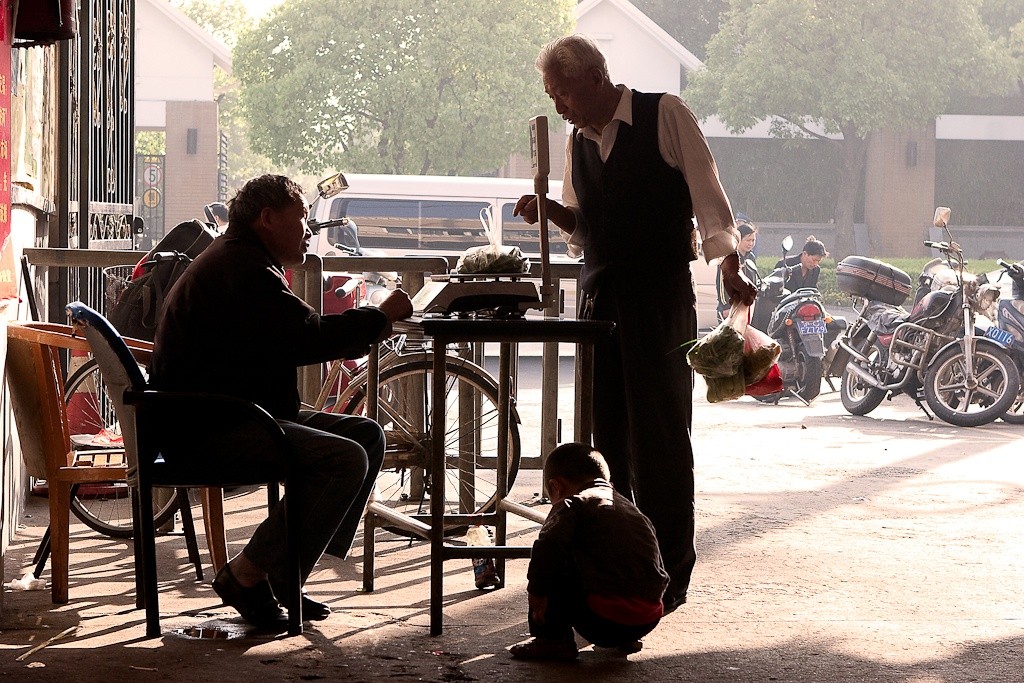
[836,254,911,306]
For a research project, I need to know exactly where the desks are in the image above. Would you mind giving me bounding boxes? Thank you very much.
[365,312,616,637]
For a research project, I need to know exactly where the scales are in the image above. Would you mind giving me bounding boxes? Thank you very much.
[409,115,554,319]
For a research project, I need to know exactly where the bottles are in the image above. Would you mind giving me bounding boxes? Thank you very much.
[466,525,501,589]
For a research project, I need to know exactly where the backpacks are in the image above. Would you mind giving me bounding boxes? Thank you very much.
[110,218,218,344]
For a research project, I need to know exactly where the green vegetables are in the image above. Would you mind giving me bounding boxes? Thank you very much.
[688,326,778,403]
[458,246,527,275]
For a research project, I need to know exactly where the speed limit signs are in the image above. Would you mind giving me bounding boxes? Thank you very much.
[143,164,162,185]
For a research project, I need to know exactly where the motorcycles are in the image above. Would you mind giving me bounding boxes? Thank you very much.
[971,258,1024,426]
[741,234,828,405]
[826,206,1021,429]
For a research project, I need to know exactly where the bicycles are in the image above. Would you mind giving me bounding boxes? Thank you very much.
[53,319,527,546]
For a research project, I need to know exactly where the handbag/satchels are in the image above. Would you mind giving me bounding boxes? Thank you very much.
[456,203,530,274]
[687,293,785,401]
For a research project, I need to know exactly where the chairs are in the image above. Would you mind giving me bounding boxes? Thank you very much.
[8,303,230,636]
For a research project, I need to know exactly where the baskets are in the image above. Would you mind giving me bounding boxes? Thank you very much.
[104,265,138,323]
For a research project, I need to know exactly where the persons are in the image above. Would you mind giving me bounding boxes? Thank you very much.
[716,224,826,325]
[149,174,414,632]
[513,33,759,617]
[205,202,229,235]
[509,442,670,662]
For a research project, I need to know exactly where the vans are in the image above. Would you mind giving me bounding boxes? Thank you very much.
[308,172,730,332]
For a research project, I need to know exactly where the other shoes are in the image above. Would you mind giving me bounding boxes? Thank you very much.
[511,639,579,659]
[211,563,289,625]
[300,592,330,615]
